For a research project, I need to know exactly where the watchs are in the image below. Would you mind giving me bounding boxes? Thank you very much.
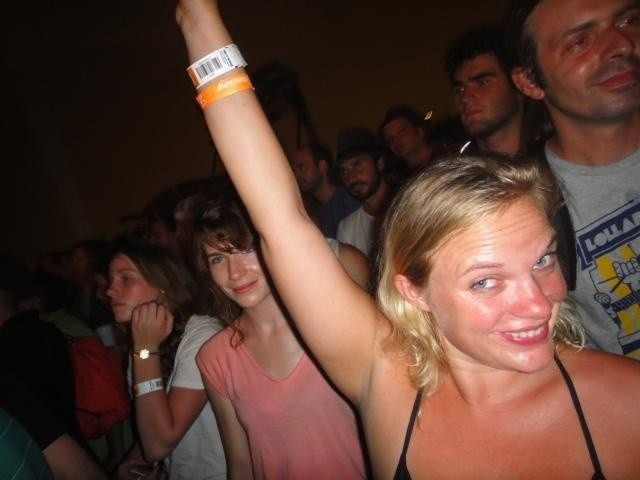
[134,347,160,360]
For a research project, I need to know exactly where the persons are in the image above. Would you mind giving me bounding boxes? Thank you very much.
[174,1,640,480]
[506,2,638,363]
[289,142,398,235]
[192,195,368,479]
[448,25,555,168]
[377,108,444,169]
[108,233,229,478]
[0,315,100,478]
[334,133,418,252]
